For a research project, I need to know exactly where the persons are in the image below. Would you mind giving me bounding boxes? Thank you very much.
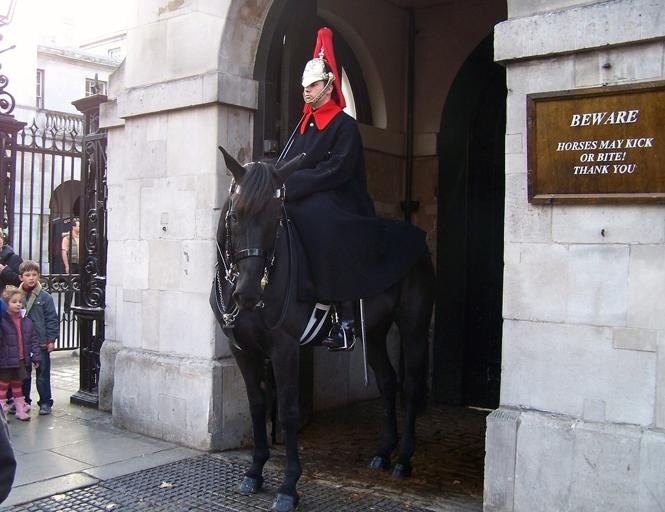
[274,28,377,350]
[12,259,58,414]
[0,284,31,420]
[0,226,31,413]
[0,296,17,502]
[61,220,80,315]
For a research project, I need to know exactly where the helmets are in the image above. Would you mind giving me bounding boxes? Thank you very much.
[302,59,341,107]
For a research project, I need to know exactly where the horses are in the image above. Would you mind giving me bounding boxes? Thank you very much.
[208,145,436,512]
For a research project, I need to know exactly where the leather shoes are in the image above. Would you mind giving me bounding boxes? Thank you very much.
[322,320,355,351]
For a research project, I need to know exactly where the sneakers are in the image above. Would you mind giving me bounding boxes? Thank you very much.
[39,403,51,415]
[7,403,30,415]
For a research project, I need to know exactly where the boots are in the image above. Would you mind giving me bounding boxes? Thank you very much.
[0,399,10,424]
[12,397,30,421]
